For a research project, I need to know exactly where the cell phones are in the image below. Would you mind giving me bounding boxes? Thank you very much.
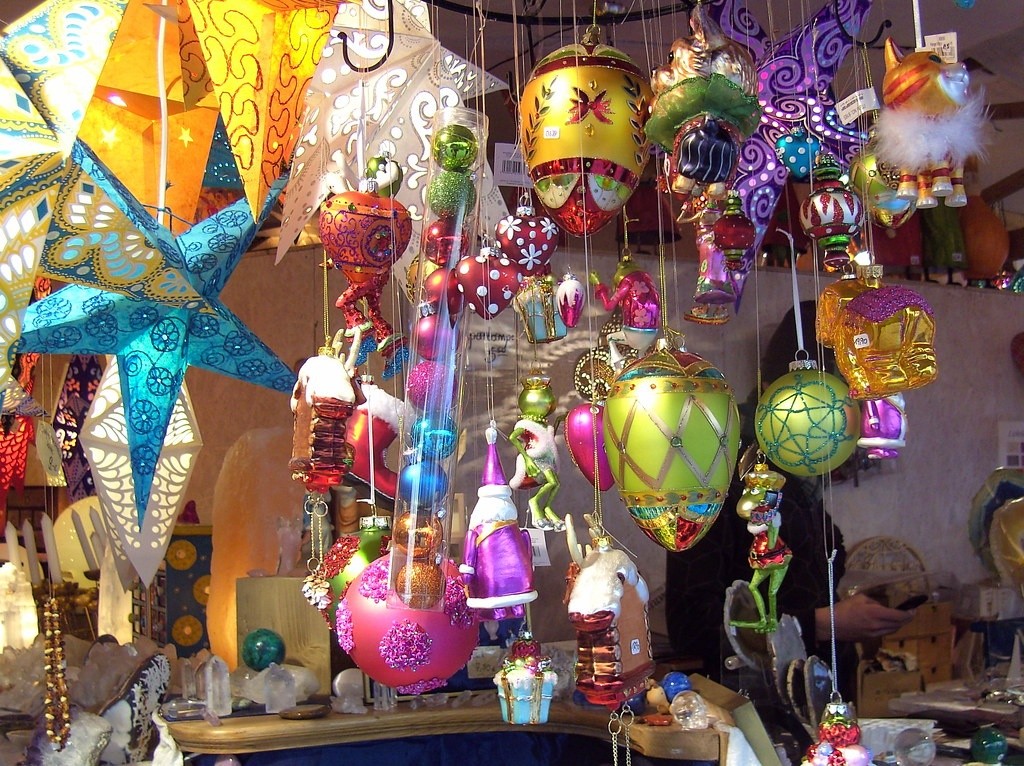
[896,596,928,612]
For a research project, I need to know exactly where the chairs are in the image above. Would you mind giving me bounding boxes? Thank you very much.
[858,655,924,719]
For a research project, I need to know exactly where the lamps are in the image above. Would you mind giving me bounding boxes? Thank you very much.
[573,307,640,402]
[77,0,202,594]
[273,0,512,264]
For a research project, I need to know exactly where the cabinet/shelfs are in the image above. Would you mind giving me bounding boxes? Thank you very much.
[130,523,212,656]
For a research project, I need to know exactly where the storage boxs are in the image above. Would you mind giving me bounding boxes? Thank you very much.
[863,602,956,687]
[964,584,1002,617]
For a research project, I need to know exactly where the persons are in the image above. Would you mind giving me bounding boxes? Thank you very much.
[665,299,919,736]
[591,258,661,365]
